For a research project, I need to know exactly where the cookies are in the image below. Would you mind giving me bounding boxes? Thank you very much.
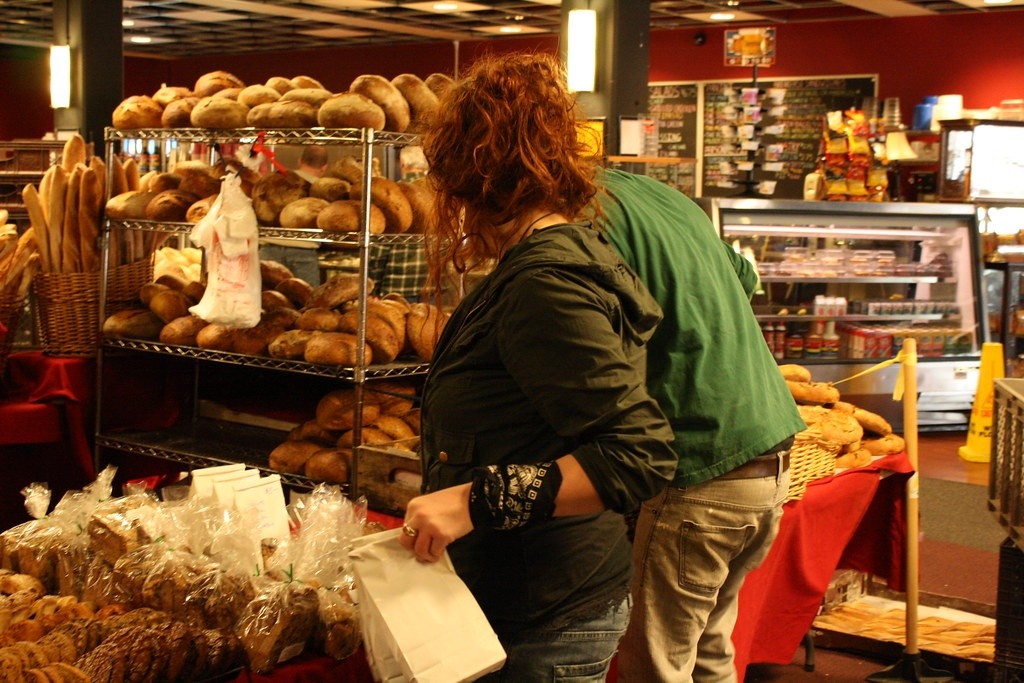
[0,607,246,683]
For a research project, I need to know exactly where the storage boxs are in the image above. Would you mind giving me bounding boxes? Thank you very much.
[837,322,972,359]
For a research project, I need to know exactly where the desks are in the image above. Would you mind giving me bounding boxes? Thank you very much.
[607,452,914,683]
[0,345,411,683]
[983,262,1024,379]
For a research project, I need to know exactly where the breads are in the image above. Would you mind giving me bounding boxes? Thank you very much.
[100,247,456,367]
[0,496,384,675]
[777,363,905,468]
[266,377,421,486]
[0,134,162,299]
[104,158,467,237]
[111,69,462,133]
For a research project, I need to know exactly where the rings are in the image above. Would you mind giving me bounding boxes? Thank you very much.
[403,523,417,537]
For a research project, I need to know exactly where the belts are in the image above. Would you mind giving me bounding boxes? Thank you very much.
[712,451,791,480]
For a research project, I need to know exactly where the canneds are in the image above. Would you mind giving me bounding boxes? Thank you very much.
[786,333,841,360]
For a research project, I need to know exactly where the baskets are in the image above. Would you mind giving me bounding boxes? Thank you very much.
[0,292,32,355]
[27,255,156,357]
[787,427,837,502]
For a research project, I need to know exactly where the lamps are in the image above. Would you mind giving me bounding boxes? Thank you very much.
[886,132,917,202]
[50,44,71,110]
[567,8,597,92]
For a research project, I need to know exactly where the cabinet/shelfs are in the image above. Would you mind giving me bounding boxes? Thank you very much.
[95,124,492,504]
[726,227,963,325]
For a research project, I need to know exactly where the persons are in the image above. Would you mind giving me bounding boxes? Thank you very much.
[398,53,676,683]
[562,154,811,683]
